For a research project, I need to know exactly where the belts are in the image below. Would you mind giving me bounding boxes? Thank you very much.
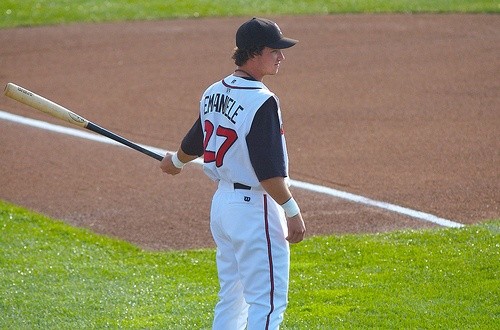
[234,182,251,190]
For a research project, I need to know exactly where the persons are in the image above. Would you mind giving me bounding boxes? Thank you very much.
[159,18,306,330]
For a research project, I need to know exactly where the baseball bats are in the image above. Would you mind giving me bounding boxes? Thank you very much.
[3,80,165,162]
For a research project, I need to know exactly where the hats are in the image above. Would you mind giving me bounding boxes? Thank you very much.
[235,18,298,49]
